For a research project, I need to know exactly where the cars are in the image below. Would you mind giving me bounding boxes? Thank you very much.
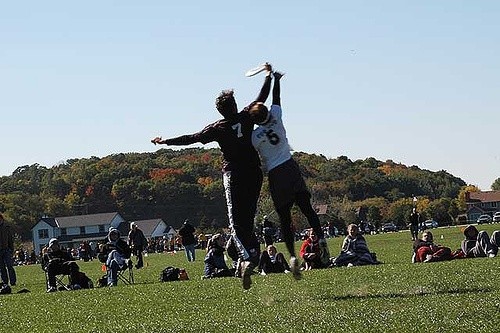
[301,220,384,240]
[384,222,398,233]
[492,212,500,223]
[477,214,493,225]
[425,220,438,229]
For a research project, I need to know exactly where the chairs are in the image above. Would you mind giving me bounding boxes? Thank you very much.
[106,249,135,285]
[45,271,72,291]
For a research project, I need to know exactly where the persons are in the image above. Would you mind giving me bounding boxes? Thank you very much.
[251,69,331,280]
[151,70,274,290]
[179,219,197,263]
[198,214,383,280]
[409,207,500,263]
[0,205,151,294]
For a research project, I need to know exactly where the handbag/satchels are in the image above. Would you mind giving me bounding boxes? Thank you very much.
[162,267,179,280]
[180,268,189,280]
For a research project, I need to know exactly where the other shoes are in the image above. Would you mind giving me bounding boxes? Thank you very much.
[347,262,353,268]
[111,260,118,275]
[319,238,330,264]
[260,271,266,276]
[109,282,117,287]
[74,284,81,289]
[291,256,301,280]
[242,261,254,290]
[423,255,433,263]
[488,253,496,257]
[47,287,57,292]
[284,270,290,274]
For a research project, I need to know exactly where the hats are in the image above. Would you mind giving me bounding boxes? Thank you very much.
[464,225,479,239]
[108,227,120,243]
[48,238,60,248]
